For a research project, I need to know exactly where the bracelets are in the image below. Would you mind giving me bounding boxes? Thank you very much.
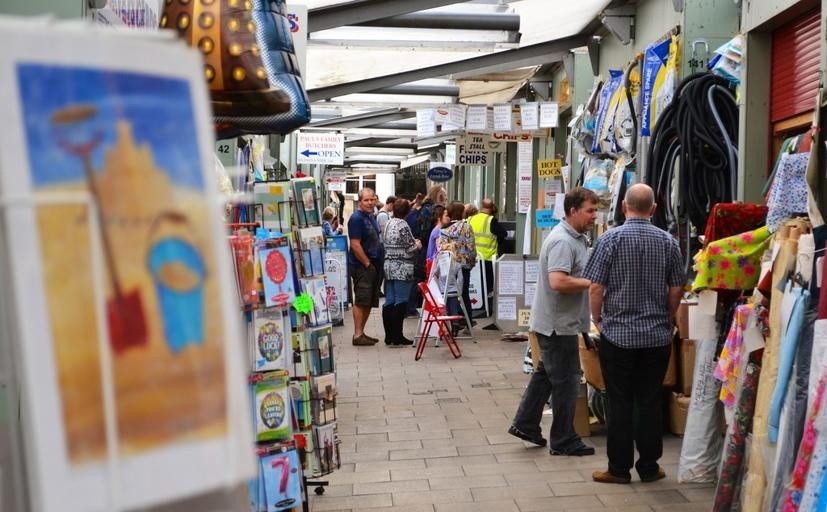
[592,317,602,323]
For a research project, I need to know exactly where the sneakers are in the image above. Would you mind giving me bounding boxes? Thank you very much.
[593,471,629,484]
[643,467,665,481]
[508,425,546,446]
[549,438,595,455]
[353,334,379,346]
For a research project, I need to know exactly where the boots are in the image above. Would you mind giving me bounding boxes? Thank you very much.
[382,303,392,345]
[392,302,414,345]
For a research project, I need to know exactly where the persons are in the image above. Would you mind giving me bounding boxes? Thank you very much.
[507,181,601,458]
[331,215,343,231]
[581,180,687,486]
[321,206,343,236]
[346,184,508,347]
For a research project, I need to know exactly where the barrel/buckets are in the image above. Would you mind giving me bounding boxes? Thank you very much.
[142,211,207,353]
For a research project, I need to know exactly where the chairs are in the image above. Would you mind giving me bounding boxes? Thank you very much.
[415,282,463,361]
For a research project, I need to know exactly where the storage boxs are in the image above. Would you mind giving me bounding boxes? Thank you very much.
[528,298,700,437]
